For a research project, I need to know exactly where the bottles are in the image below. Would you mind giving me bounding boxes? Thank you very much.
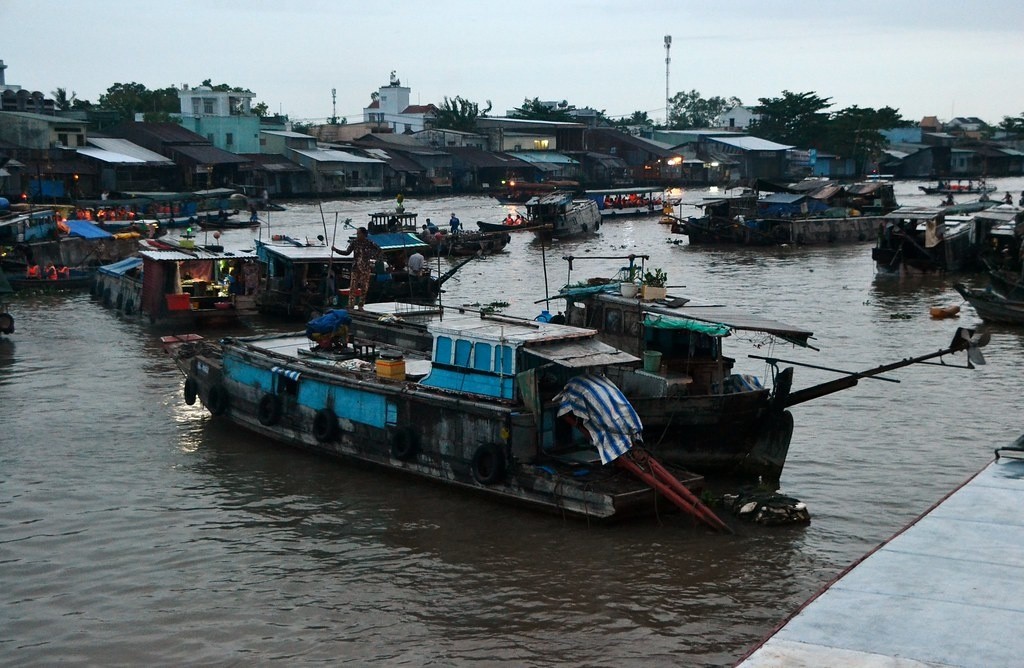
[538,310,552,322]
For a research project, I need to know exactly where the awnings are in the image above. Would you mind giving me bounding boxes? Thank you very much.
[172,146,254,163]
[77,138,177,167]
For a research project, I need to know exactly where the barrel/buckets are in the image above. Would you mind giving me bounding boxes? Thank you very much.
[644,351,663,371]
[165,295,190,310]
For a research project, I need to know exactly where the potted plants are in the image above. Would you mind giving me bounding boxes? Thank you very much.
[619,254,639,298]
[644,267,668,300]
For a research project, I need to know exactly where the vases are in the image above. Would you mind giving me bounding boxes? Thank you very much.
[396,208,405,214]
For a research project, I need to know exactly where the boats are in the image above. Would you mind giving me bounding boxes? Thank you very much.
[523,249,815,490]
[3,174,1024,332]
[161,293,705,525]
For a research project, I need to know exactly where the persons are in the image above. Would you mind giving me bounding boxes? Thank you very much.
[218,207,224,223]
[449,212,459,233]
[421,218,438,236]
[969,179,973,191]
[332,227,378,311]
[1001,191,1012,203]
[502,214,524,225]
[77,207,135,221]
[945,193,954,205]
[101,191,109,200]
[262,188,268,204]
[977,179,985,191]
[223,267,242,295]
[389,216,399,227]
[159,205,179,213]
[979,192,989,202]
[28,260,69,279]
[945,180,951,190]
[605,194,661,208]
[250,204,257,224]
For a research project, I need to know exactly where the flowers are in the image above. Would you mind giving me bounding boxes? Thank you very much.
[395,194,404,208]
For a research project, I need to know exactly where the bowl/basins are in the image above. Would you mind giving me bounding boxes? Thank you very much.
[214,303,231,309]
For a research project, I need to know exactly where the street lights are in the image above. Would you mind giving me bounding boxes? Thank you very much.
[664,35,671,120]
